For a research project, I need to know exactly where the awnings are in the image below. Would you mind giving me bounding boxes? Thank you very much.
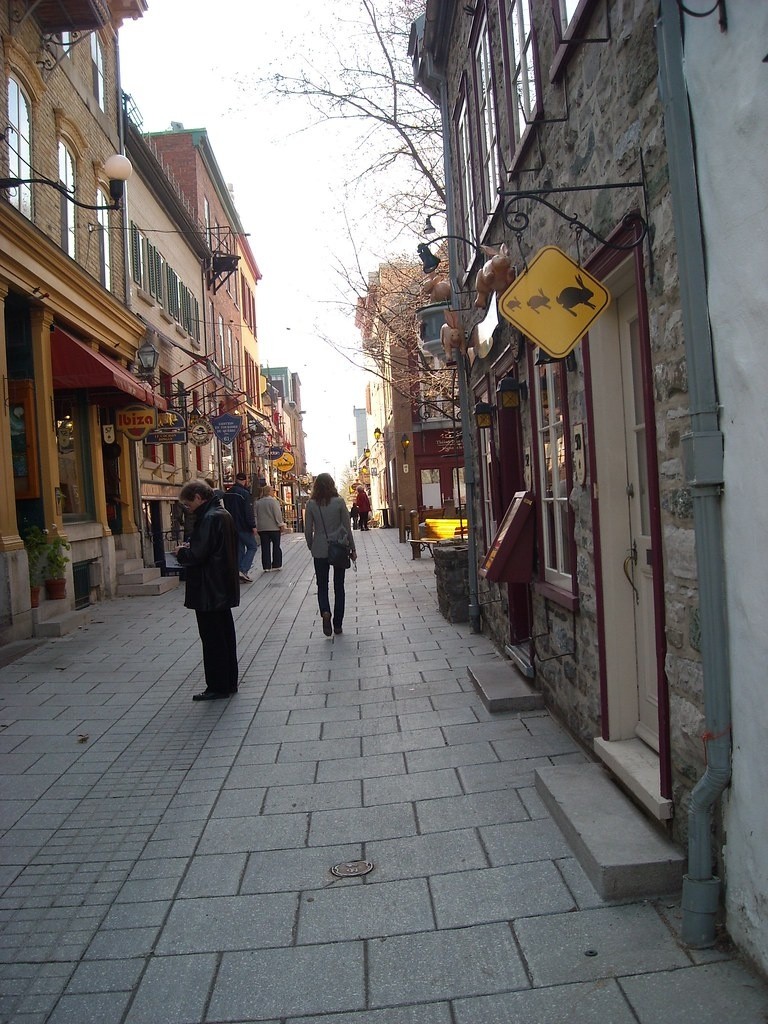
[49,322,168,427]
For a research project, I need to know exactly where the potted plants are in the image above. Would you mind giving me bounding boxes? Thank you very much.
[41,537,73,600]
[21,525,48,608]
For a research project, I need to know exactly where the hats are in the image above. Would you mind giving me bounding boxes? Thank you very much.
[236,473,247,481]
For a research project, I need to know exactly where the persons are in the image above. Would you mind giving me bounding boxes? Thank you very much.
[169,482,241,701]
[355,484,371,531]
[203,473,258,582]
[253,486,285,572]
[305,473,357,637]
[350,503,359,529]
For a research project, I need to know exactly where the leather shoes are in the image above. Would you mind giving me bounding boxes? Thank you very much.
[229,688,238,693]
[193,691,229,700]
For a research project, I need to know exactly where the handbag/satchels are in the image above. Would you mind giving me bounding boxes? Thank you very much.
[327,540,350,568]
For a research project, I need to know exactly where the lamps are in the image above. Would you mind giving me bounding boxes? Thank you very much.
[400,433,411,461]
[417,236,484,274]
[0,155,133,211]
[534,347,577,372]
[424,209,446,234]
[472,398,497,429]
[349,427,384,493]
[496,370,528,410]
[138,338,159,371]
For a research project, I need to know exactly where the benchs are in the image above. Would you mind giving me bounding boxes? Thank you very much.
[407,518,469,561]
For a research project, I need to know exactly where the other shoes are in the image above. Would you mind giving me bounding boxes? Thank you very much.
[265,569,270,571]
[323,612,332,636]
[271,567,282,570]
[334,627,342,633]
[239,571,253,582]
